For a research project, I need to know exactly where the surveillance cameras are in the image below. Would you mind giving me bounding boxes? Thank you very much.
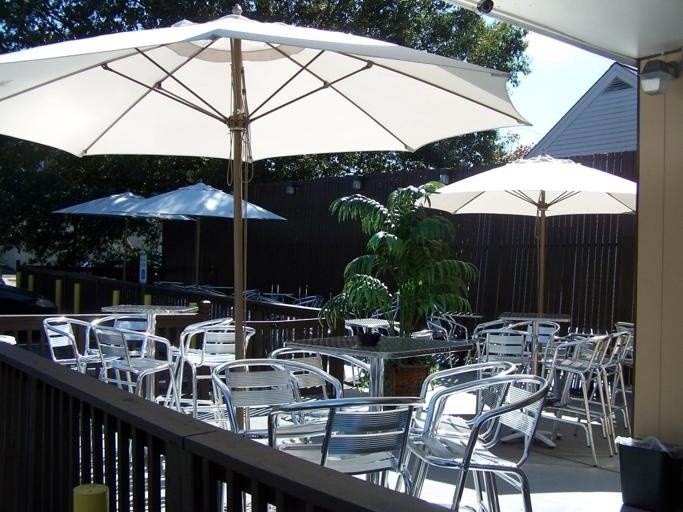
[477,0,493,13]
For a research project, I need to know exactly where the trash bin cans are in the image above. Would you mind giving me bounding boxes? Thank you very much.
[615,436,683,512]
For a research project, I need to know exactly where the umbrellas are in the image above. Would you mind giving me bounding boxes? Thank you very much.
[0,13,534,164]
[52,191,198,223]
[110,181,288,224]
[413,154,638,217]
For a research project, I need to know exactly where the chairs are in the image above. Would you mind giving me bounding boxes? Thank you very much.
[269,393,414,497]
[91,321,181,412]
[162,279,330,310]
[535,336,613,468]
[472,330,538,376]
[163,324,255,421]
[168,314,233,410]
[43,315,117,378]
[394,359,517,512]
[550,332,612,463]
[208,358,342,457]
[267,348,371,406]
[499,318,560,362]
[423,316,467,342]
[613,321,635,372]
[391,372,550,512]
[566,331,633,453]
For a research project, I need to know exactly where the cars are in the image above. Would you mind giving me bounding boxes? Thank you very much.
[0,277,57,315]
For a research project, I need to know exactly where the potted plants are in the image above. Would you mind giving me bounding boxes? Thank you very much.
[318,180,483,399]
